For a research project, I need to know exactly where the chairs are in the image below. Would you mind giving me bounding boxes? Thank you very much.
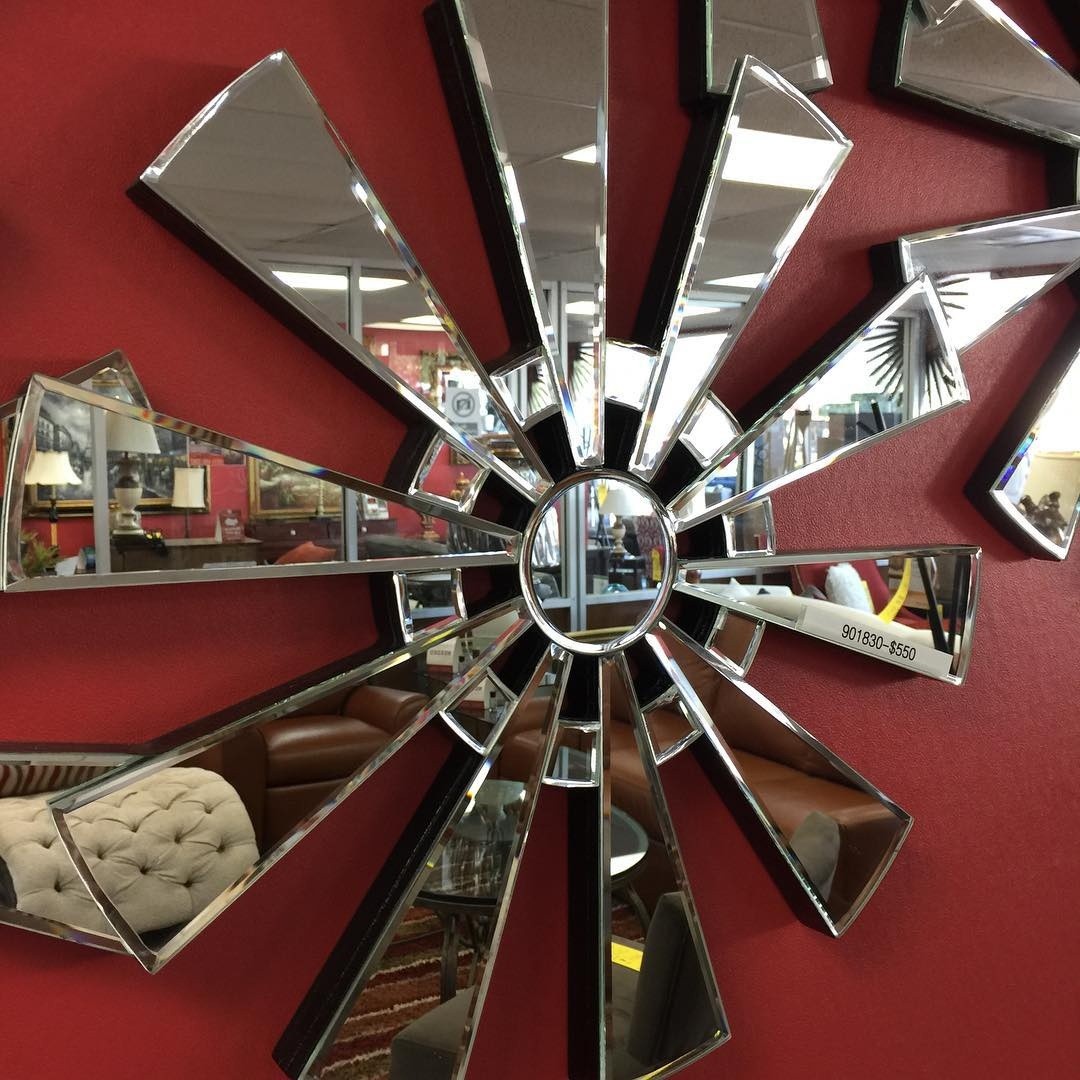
[790,559,929,630]
[224,684,431,852]
[390,889,717,1080]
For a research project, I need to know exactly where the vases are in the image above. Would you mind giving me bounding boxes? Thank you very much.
[613,514,627,554]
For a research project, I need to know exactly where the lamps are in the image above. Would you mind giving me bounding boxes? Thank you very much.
[24,448,82,557]
[171,467,208,538]
[104,409,162,536]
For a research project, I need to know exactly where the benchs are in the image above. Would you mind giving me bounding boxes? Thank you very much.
[500,696,903,923]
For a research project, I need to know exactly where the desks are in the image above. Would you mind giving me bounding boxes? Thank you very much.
[110,537,263,572]
[608,555,646,590]
[410,780,650,1003]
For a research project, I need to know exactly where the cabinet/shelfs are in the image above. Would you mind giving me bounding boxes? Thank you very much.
[243,519,398,541]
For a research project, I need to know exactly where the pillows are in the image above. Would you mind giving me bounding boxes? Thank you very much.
[825,562,873,614]
[274,542,336,562]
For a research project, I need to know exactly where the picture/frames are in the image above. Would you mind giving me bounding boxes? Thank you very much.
[24,392,192,519]
[245,455,343,520]
